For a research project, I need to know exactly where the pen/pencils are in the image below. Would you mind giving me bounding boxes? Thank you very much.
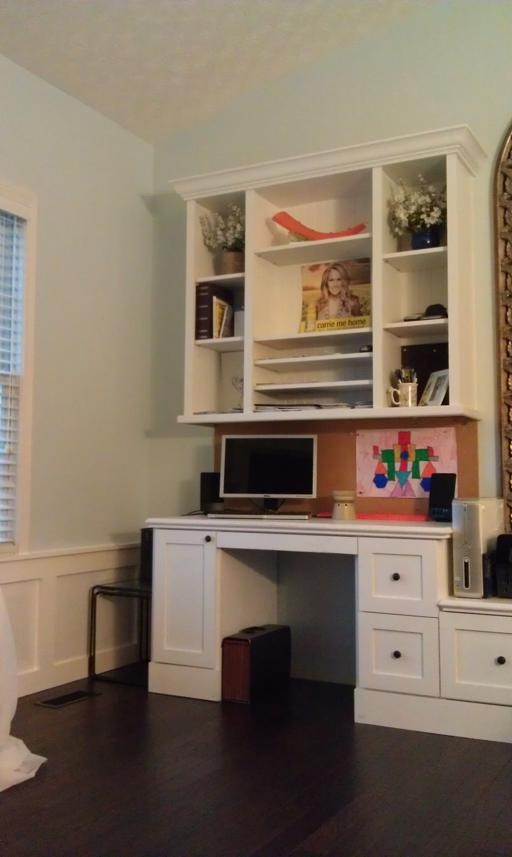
[396,368,418,383]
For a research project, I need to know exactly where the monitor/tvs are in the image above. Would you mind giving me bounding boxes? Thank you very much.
[219,434,319,511]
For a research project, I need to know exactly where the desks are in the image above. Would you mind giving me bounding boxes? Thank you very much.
[135,511,510,747]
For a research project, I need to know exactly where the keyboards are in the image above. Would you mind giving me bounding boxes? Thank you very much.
[207,508,312,521]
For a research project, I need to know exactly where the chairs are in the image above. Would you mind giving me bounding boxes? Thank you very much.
[87,529,153,686]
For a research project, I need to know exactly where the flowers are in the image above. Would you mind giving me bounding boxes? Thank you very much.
[198,202,246,254]
[383,169,447,237]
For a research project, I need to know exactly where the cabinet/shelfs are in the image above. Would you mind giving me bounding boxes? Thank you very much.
[163,122,492,426]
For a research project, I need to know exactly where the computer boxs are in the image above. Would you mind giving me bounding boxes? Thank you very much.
[451,497,506,598]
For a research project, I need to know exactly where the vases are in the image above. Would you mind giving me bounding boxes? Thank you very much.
[411,227,440,248]
[220,252,244,274]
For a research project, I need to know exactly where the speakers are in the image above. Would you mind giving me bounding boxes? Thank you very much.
[495,533,512,599]
[428,472,458,521]
[199,472,224,512]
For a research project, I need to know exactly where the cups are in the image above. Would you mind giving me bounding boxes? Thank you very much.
[390,383,419,407]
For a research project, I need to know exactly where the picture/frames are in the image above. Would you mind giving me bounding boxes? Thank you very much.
[418,369,450,406]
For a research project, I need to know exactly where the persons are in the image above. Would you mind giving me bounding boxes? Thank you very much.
[313,264,360,322]
